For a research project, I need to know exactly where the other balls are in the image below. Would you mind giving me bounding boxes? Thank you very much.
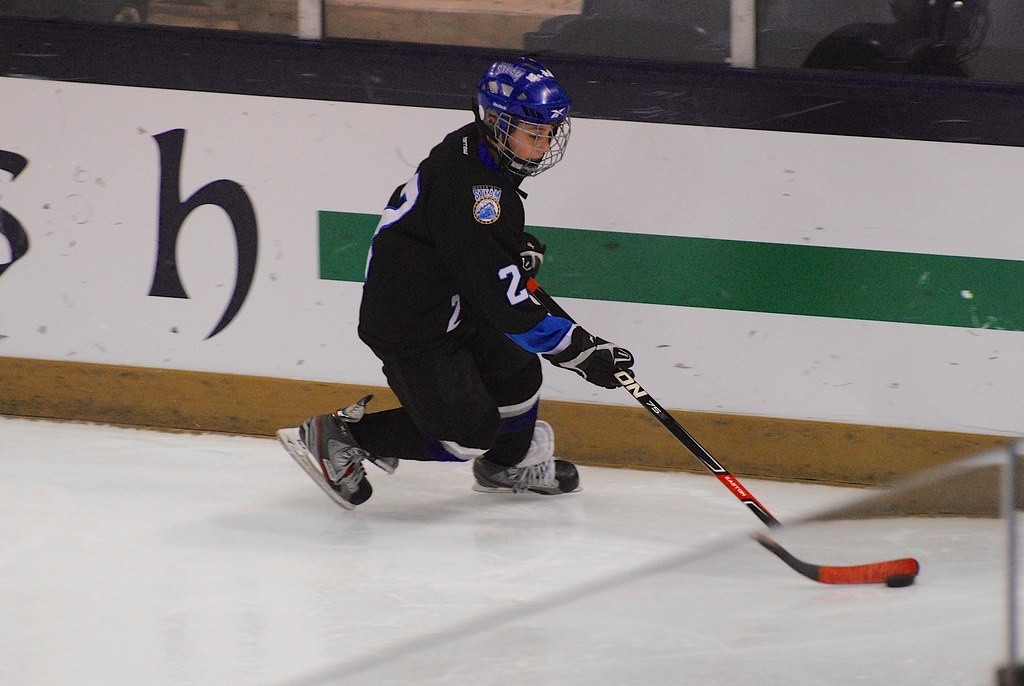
[886,574,914,587]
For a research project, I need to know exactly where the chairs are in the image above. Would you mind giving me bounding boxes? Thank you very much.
[524,0,983,81]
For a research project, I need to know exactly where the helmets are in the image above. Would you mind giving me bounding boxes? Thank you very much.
[477,56,570,143]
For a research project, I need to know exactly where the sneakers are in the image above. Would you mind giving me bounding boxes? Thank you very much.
[469,418,583,496]
[275,394,374,511]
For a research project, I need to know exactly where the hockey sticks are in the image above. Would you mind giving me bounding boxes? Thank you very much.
[525,278,920,584]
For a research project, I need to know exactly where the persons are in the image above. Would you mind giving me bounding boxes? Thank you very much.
[275,55,635,510]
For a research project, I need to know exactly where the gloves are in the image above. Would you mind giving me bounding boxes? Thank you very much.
[541,324,636,389]
[519,230,547,278]
